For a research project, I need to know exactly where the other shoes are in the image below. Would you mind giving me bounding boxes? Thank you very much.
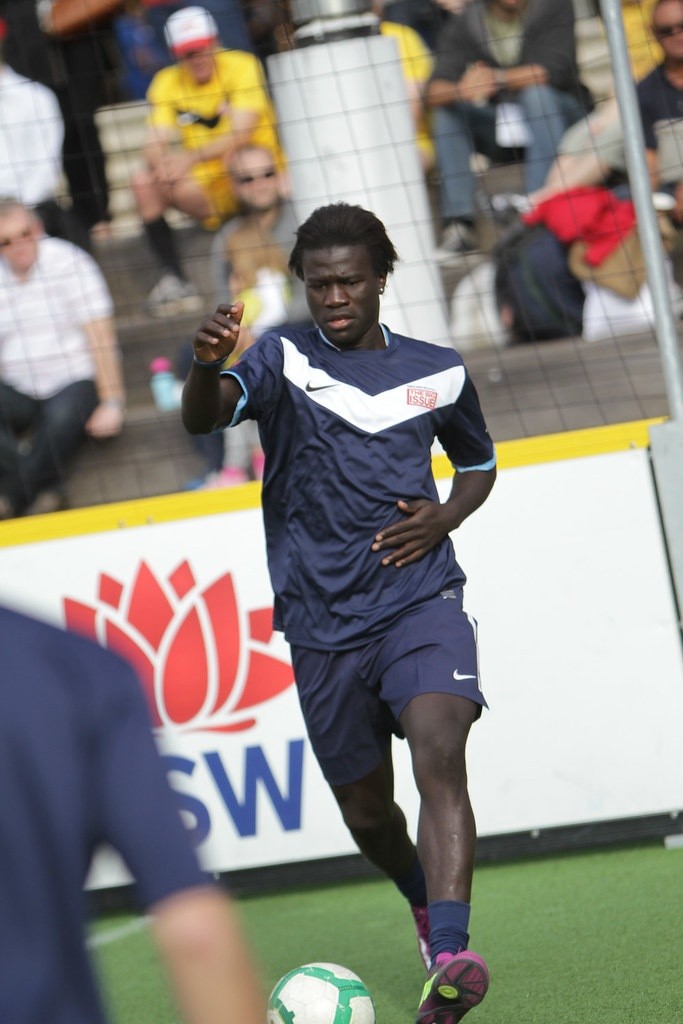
[435,220,486,269]
[489,193,532,215]
[148,276,204,321]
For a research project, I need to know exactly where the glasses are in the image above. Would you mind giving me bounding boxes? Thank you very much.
[237,170,276,182]
[0,229,34,246]
[651,20,683,40]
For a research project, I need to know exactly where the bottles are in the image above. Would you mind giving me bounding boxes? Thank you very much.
[148,357,180,414]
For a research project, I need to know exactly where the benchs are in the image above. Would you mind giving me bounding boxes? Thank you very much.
[60,0,683,516]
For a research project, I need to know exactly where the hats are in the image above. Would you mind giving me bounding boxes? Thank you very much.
[162,5,219,59]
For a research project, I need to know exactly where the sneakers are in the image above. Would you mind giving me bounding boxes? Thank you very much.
[414,945,490,1024]
[410,904,432,973]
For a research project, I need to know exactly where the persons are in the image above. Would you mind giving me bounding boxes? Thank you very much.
[370,1,683,347]
[0,0,310,519]
[182,202,498,1024]
[0,606,264,1024]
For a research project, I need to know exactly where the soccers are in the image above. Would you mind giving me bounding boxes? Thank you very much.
[264,961,377,1024]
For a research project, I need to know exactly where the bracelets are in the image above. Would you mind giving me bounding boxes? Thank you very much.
[192,353,228,365]
[495,70,504,90]
